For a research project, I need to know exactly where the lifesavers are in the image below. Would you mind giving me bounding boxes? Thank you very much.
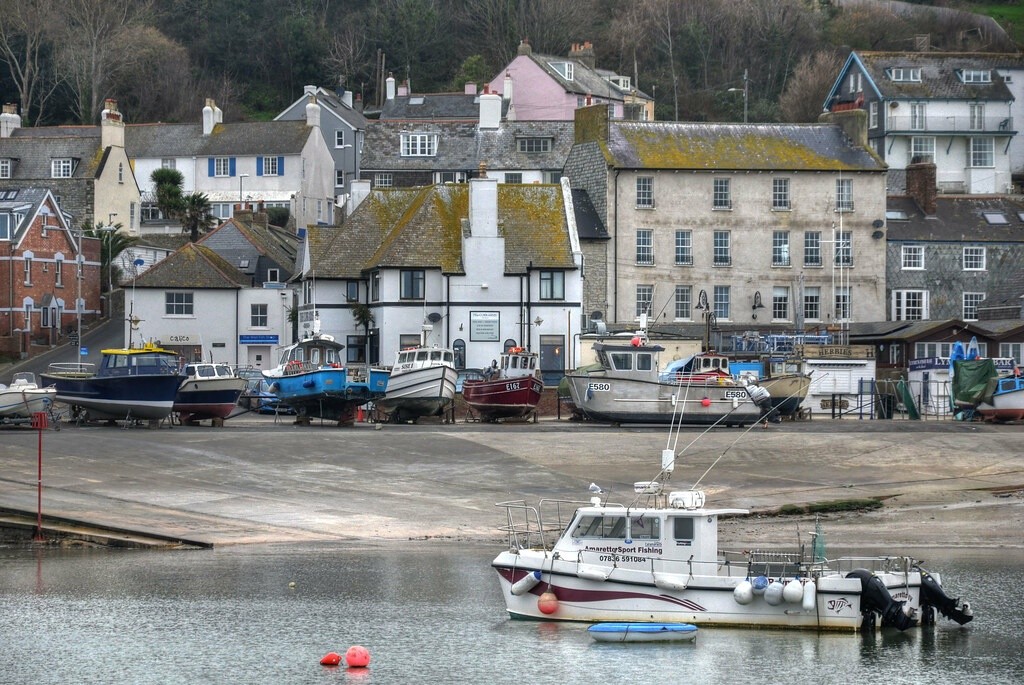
[510,347,521,352]
[287,360,303,373]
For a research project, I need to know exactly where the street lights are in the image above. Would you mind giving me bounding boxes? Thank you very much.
[43,225,117,369]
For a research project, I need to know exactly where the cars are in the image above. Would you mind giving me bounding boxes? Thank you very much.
[232,366,296,414]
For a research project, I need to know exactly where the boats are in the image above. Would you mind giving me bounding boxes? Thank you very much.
[40,299,190,421]
[754,372,811,415]
[374,344,459,417]
[173,360,249,417]
[260,334,392,411]
[491,358,978,632]
[975,369,1024,424]
[0,370,58,419]
[588,621,699,643]
[460,348,545,419]
[563,331,765,423]
